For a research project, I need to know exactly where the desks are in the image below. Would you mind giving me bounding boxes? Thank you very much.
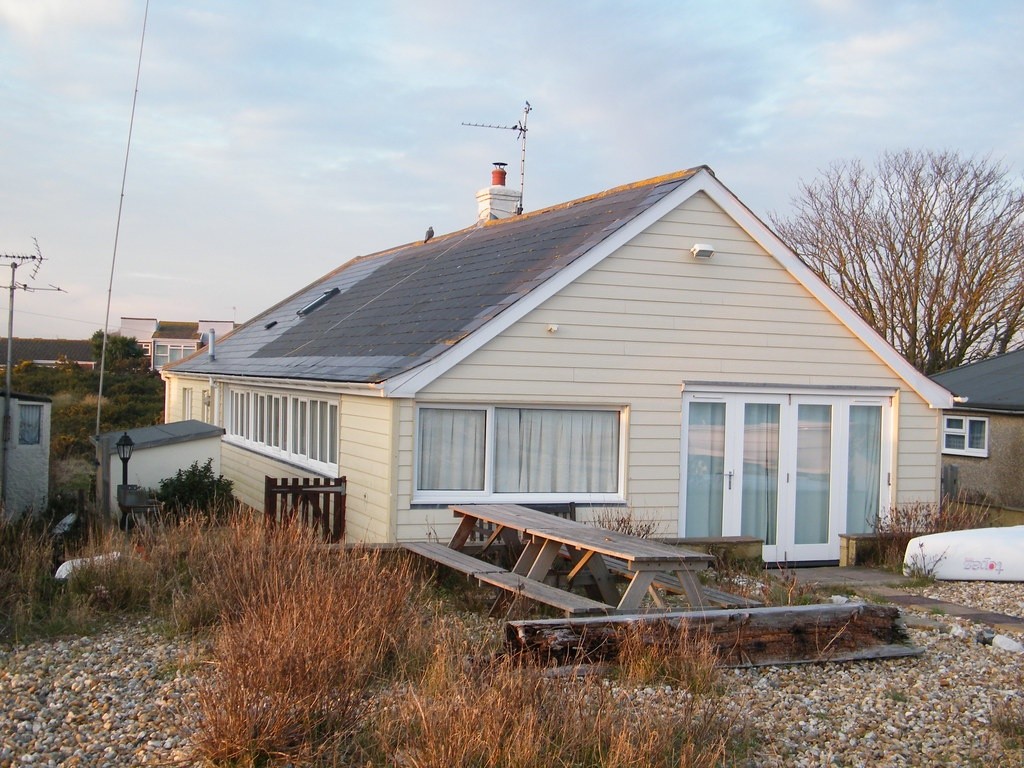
[434,505,590,618]
[504,527,715,632]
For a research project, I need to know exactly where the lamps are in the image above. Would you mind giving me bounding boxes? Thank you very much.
[690,243,715,260]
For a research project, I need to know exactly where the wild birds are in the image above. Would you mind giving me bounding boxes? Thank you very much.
[424,226,434,243]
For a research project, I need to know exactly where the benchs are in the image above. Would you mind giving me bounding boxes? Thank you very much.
[837,532,923,567]
[558,542,767,637]
[400,541,509,619]
[473,572,617,624]
